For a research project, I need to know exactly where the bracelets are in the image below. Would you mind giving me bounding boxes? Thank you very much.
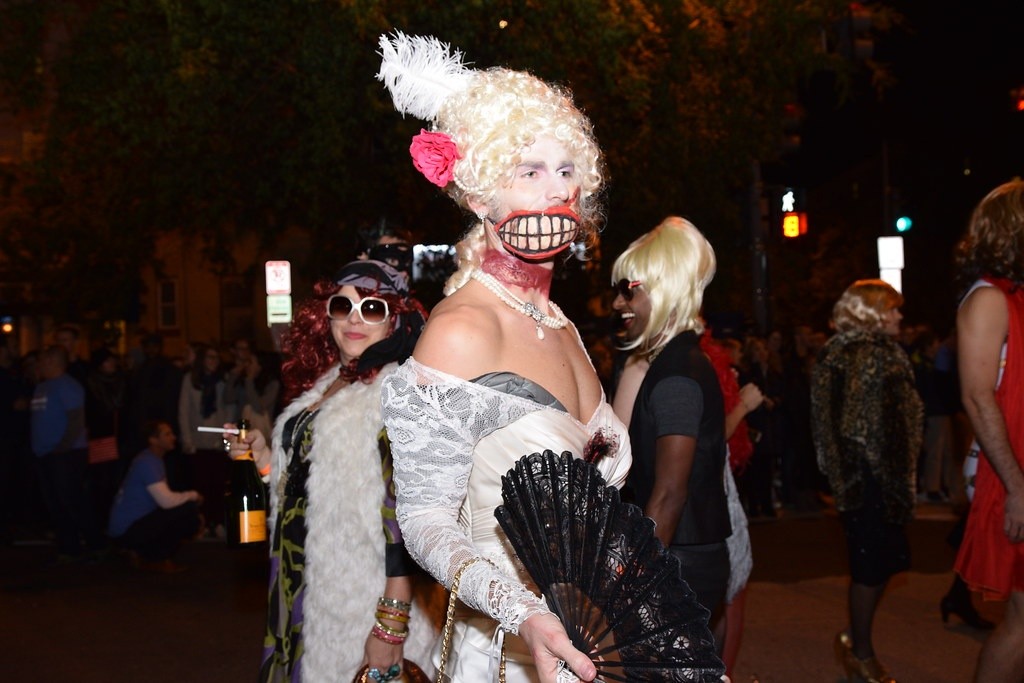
[373,597,412,644]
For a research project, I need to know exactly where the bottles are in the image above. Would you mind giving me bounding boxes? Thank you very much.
[224,419,267,551]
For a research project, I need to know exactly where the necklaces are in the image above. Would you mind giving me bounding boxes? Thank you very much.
[471,267,568,338]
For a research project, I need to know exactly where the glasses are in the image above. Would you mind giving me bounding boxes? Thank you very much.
[326,294,389,324]
[611,278,641,301]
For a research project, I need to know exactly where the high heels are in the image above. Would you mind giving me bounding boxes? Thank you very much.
[833,628,896,683]
[941,596,995,629]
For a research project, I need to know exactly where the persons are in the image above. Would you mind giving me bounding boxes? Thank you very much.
[941,182,1024,683]
[223,260,448,683]
[611,216,733,659]
[379,36,730,683]
[1,225,430,574]
[577,279,975,683]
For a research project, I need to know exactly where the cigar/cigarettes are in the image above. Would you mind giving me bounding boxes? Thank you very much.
[198,427,239,435]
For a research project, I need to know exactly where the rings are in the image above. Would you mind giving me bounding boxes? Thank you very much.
[223,439,230,451]
[368,664,400,683]
